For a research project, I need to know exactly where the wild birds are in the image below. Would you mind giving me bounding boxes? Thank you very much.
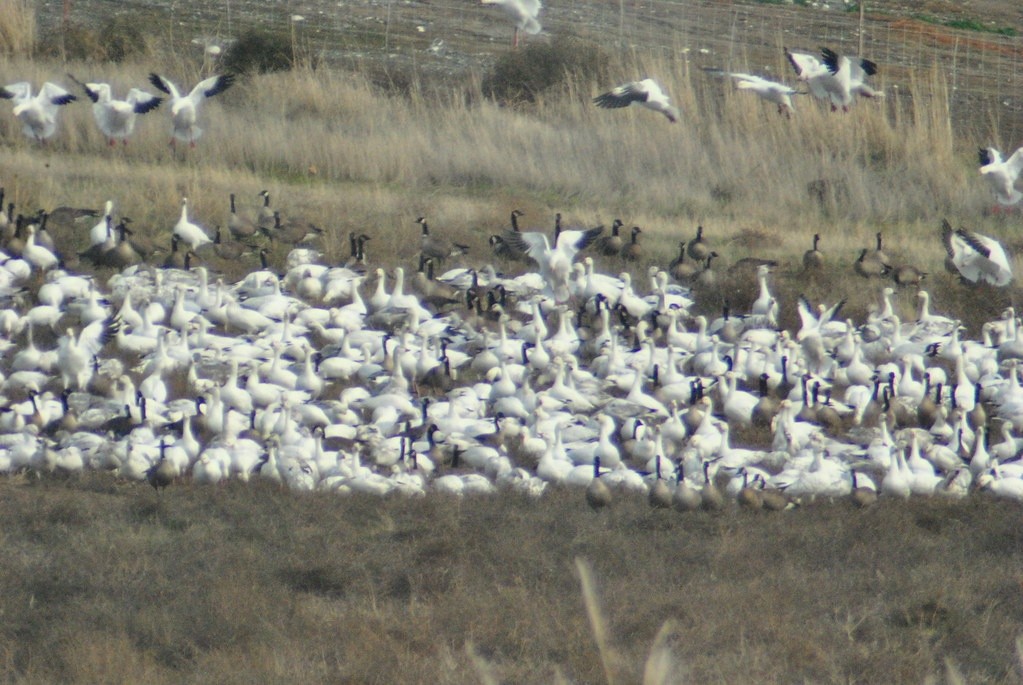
[732,43,885,115]
[977,145,1023,208]
[146,70,237,150]
[1,188,1023,507]
[0,80,83,145]
[473,1,547,40]
[592,75,685,123]
[66,71,167,148]
